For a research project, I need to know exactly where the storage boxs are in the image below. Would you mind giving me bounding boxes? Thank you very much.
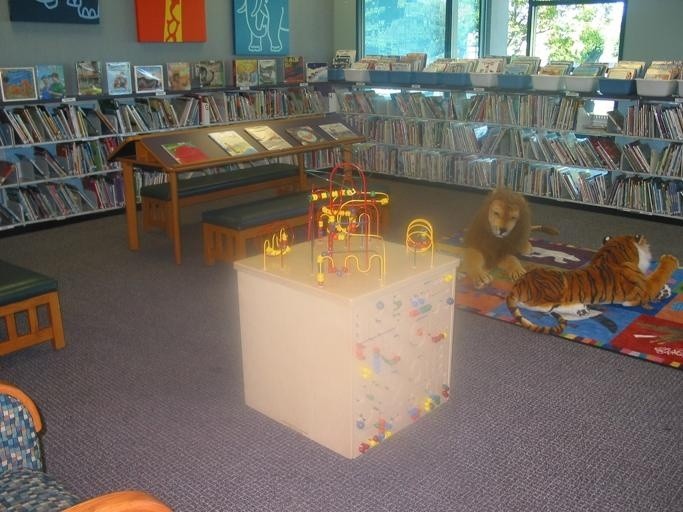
[325,66,529,90]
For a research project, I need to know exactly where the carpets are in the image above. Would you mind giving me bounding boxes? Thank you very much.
[435,231,683,370]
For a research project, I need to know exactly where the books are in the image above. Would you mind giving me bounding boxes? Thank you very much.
[0,49,683,227]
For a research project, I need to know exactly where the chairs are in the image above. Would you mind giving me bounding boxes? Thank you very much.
[0,385,174,512]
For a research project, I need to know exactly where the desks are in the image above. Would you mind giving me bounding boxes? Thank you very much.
[233,232,461,460]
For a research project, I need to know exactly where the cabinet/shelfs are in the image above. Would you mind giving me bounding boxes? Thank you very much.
[0,82,357,233]
[333,81,683,220]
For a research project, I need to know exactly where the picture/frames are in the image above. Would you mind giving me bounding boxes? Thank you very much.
[0,66,38,102]
[133,65,165,94]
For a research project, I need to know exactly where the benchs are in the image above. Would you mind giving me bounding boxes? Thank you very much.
[0,260,65,361]
[139,162,308,238]
[199,187,387,269]
